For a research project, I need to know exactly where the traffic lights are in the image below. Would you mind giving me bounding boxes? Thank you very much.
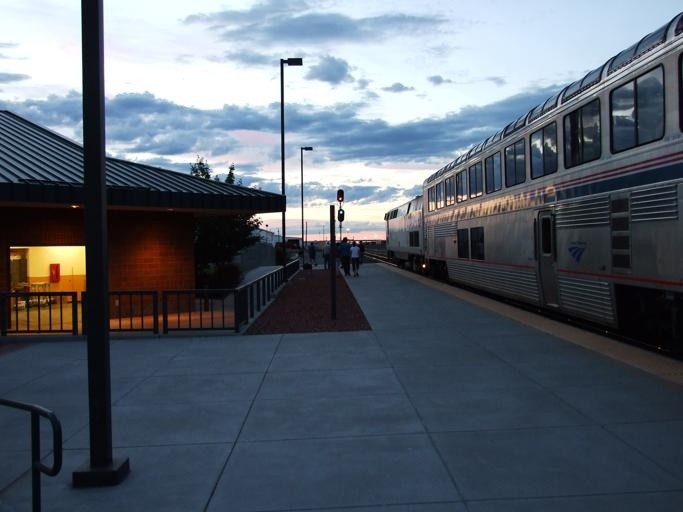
[337,189,345,223]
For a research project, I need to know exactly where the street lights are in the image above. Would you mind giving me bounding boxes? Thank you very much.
[300,146,314,248]
[279,55,304,242]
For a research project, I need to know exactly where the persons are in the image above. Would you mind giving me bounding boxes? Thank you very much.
[336,238,365,277]
[322,242,331,270]
[308,242,318,266]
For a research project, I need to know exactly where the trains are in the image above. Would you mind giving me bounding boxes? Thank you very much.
[382,8,682,356]
